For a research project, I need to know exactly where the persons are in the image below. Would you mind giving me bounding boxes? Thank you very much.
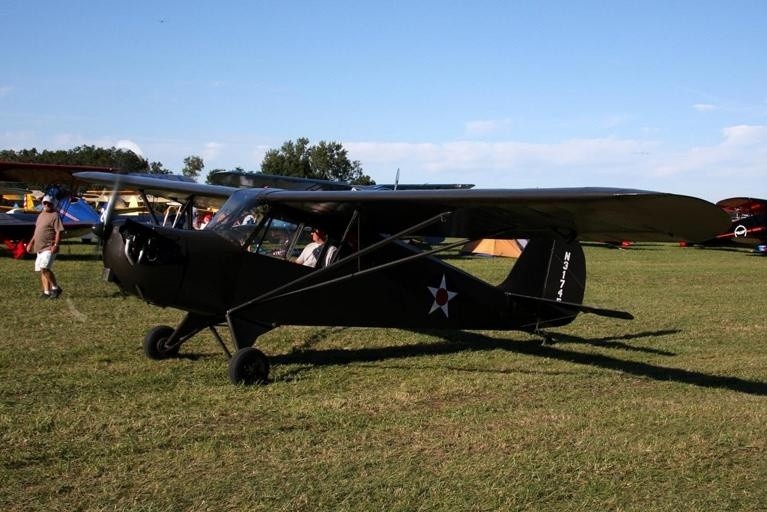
[192,211,258,253]
[294,226,339,271]
[25,194,65,300]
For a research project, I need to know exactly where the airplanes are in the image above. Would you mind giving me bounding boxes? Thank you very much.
[681,194,767,257]
[68,164,736,389]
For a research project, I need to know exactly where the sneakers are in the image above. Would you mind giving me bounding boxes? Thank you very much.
[38,286,63,299]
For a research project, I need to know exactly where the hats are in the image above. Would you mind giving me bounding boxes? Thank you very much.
[42,194,55,206]
